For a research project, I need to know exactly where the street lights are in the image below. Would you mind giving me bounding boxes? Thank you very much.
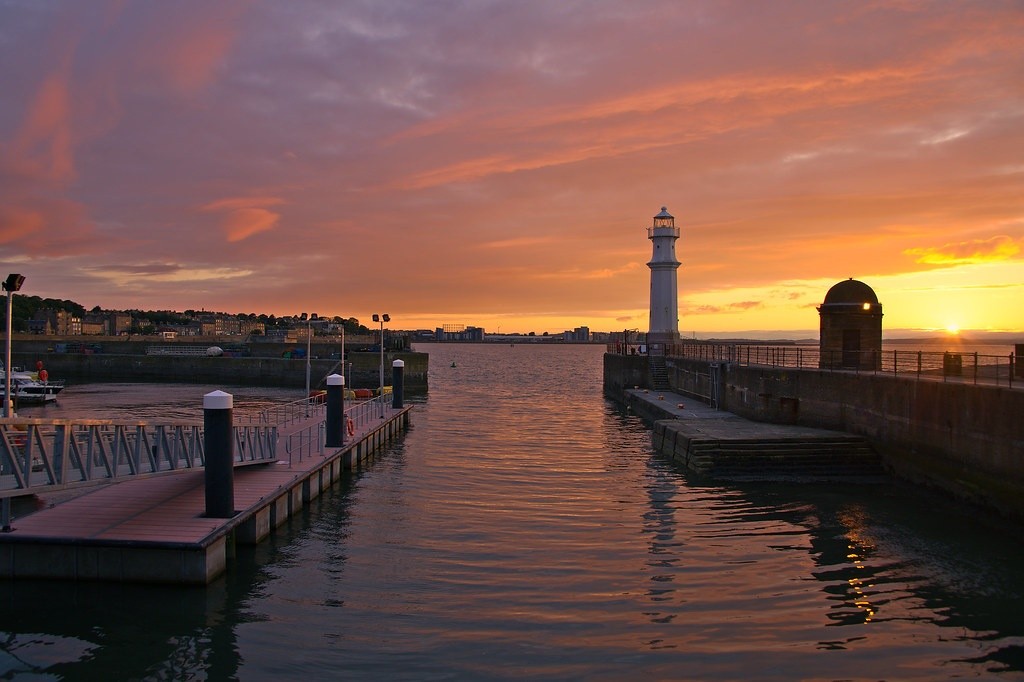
[305,320,325,413]
[2,273,27,420]
[373,313,390,417]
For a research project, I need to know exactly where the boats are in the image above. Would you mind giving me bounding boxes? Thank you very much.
[1,358,63,405]
[450,361,456,367]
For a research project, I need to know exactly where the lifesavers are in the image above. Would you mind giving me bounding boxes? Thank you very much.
[347,419,355,436]
